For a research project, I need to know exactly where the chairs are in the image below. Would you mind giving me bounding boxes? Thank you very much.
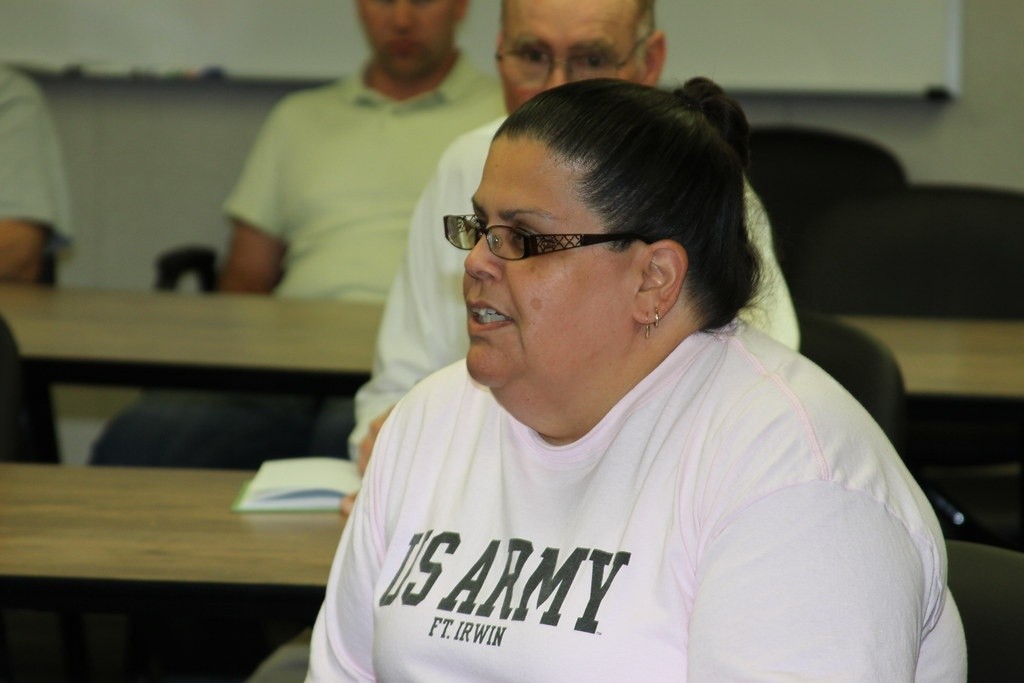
[745,122,1024,326]
[797,306,909,449]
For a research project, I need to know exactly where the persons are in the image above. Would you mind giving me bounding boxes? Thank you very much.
[0,0,801,683]
[303,76,969,682]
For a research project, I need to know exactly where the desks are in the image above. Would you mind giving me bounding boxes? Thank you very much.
[0,460,343,616]
[0,276,1024,416]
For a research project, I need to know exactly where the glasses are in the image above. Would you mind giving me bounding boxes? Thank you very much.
[442,212,650,260]
[494,34,644,85]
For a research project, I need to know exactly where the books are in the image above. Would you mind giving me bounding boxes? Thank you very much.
[229,456,363,514]
